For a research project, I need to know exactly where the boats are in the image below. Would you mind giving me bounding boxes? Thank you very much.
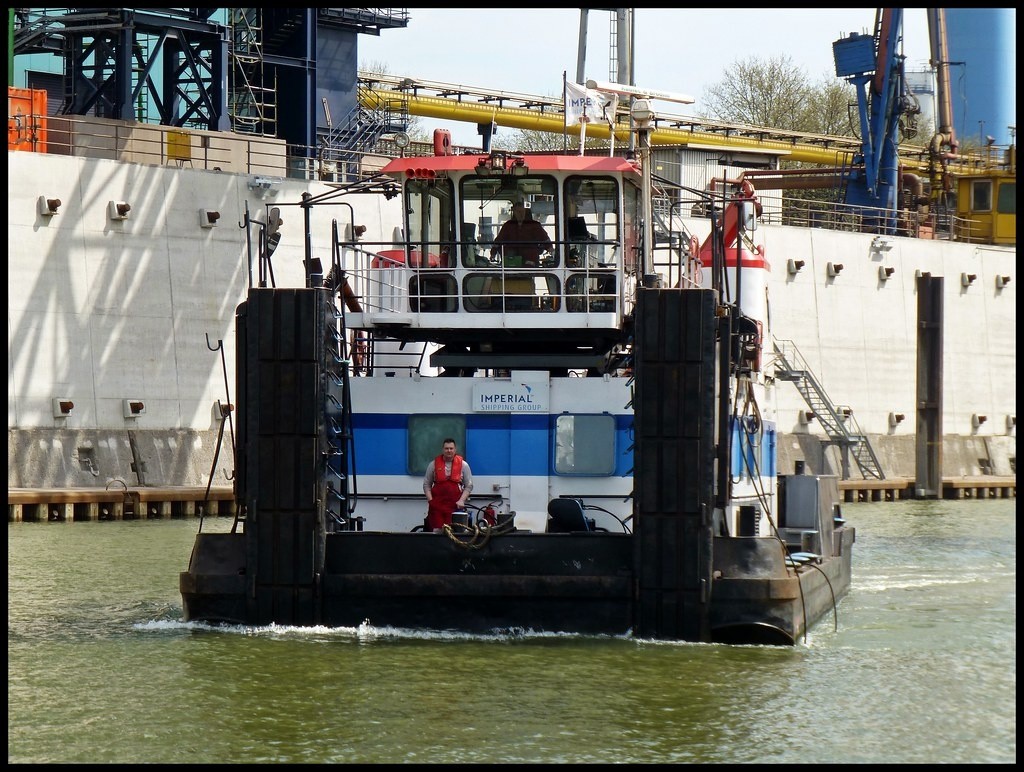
[179,71,858,646]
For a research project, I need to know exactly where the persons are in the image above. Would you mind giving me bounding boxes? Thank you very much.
[423,438,473,532]
[490,202,553,268]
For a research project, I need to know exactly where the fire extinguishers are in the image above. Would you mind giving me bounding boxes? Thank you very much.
[484,502,497,527]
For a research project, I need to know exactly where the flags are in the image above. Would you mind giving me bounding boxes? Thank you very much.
[565,79,619,127]
